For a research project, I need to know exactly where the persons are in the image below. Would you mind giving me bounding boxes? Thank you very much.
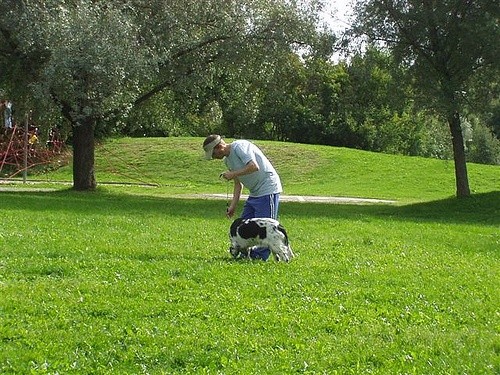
[202,135,283,261]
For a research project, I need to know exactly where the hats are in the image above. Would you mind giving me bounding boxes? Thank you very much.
[203,135,221,160]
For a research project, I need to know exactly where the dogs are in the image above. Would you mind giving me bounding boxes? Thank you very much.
[229,218,295,263]
